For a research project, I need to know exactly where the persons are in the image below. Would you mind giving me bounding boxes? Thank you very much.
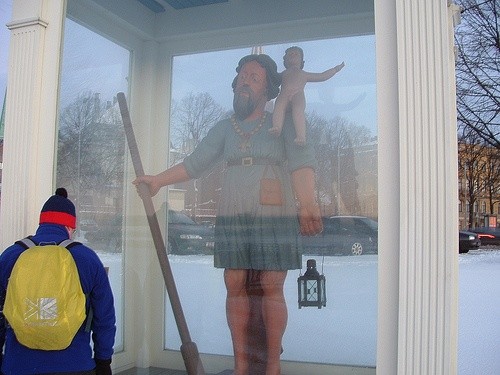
[0,188,117,375]
[268,46,346,147]
[132,53,324,375]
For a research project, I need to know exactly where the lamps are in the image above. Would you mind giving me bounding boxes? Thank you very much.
[294,221,327,310]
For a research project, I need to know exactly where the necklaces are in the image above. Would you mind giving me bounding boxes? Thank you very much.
[230,114,266,146]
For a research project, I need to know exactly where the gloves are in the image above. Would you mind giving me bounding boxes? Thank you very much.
[94,358,112,375]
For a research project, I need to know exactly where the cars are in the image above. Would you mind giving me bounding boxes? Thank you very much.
[301,215,378,256]
[106,210,211,254]
[468,227,500,245]
[459,230,481,253]
[80,219,104,242]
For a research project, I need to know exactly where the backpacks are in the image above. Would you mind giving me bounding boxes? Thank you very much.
[1,236,84,351]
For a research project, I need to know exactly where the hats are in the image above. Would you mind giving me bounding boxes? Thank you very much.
[39,187,77,230]
